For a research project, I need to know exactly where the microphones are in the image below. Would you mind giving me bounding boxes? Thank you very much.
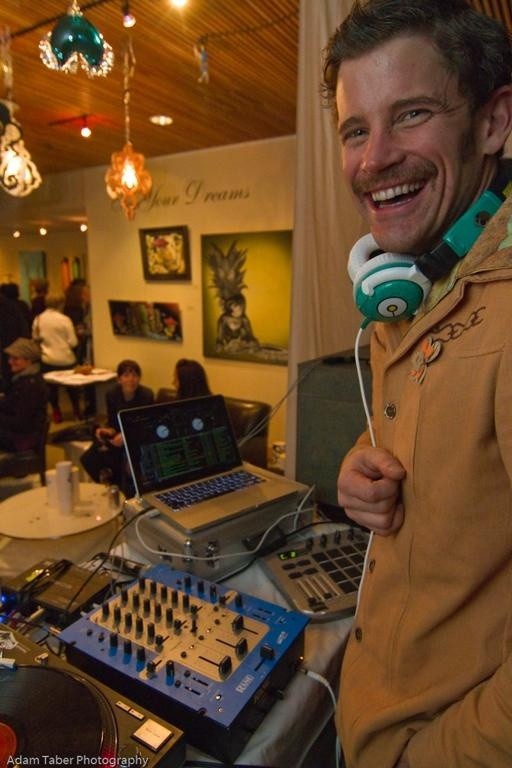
[100,551,153,578]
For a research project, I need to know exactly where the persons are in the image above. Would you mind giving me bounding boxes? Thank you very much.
[317,2,512,767]
[0,279,95,395]
[112,301,183,342]
[32,288,86,423]
[80,359,155,485]
[217,294,259,354]
[0,337,50,479]
[171,358,215,402]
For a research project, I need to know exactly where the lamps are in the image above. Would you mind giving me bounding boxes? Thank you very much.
[103,2,153,228]
[37,0,116,77]
[3,6,41,202]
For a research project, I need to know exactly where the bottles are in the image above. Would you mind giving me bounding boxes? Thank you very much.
[108,484,119,511]
[45,461,80,514]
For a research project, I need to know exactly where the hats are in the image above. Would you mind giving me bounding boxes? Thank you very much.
[4,336,43,359]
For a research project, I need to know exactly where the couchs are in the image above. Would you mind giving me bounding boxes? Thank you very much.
[110,388,270,485]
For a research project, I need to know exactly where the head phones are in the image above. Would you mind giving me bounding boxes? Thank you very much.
[346,156,512,320]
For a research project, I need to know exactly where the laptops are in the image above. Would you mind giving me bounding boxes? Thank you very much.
[117,394,299,535]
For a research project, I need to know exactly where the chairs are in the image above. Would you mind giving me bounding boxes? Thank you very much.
[3,375,55,485]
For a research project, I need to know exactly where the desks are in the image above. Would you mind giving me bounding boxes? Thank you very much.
[38,363,121,422]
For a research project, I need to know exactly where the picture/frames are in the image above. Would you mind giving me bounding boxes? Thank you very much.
[200,229,295,368]
[138,221,192,284]
